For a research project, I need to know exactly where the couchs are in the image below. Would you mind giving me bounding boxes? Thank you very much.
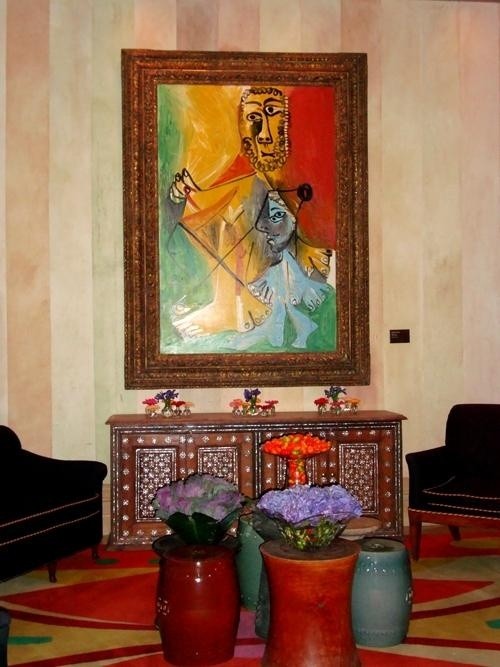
[0,425,108,584]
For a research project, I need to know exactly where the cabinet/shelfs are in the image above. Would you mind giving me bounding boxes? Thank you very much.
[105,411,408,551]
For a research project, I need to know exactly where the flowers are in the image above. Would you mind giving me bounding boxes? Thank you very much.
[259,433,332,459]
[153,474,248,545]
[142,390,195,411]
[315,385,360,407]
[229,386,278,412]
[259,485,364,551]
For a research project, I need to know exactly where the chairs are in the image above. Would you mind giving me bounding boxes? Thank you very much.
[405,403,500,562]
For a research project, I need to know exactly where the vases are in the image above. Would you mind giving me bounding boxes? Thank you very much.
[317,405,357,415]
[146,405,190,417]
[234,407,275,417]
[286,460,308,487]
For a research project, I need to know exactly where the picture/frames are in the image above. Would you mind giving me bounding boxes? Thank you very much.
[120,48,370,390]
